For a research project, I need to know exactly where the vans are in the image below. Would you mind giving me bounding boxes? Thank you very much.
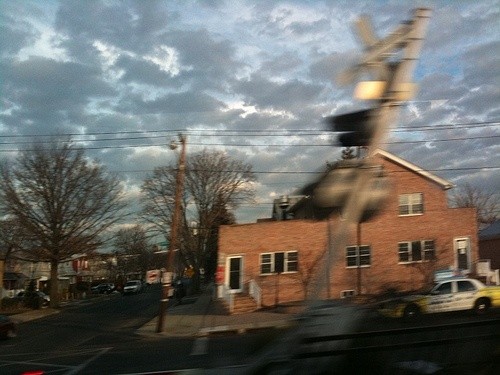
[123,280,143,295]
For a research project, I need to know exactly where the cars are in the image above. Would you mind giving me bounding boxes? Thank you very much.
[72,277,131,295]
[373,275,500,325]
[14,289,51,309]
